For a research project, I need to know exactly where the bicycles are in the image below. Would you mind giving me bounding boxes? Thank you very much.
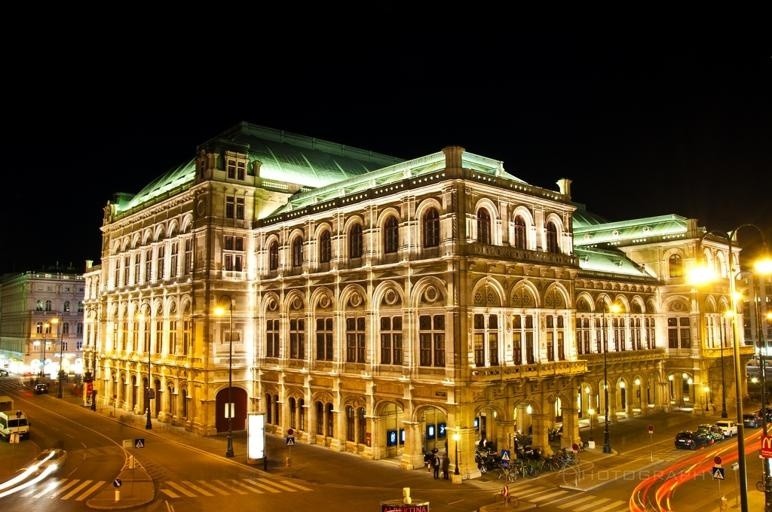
[494,493,519,508]
[493,456,574,482]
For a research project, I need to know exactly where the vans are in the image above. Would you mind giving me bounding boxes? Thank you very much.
[67,373,81,383]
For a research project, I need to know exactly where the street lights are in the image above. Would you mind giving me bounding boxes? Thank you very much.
[214,294,234,456]
[50,317,64,398]
[733,261,772,434]
[136,303,152,430]
[602,304,621,453]
[696,224,772,512]
[85,307,98,381]
[719,311,733,418]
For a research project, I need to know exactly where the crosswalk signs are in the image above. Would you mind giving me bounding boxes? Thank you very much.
[135,439,144,448]
[501,450,510,461]
[286,436,295,446]
[712,466,725,480]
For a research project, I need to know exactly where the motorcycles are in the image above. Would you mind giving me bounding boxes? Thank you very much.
[475,441,500,474]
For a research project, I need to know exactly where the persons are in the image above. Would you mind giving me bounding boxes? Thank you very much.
[433,454,440,480]
[443,453,450,480]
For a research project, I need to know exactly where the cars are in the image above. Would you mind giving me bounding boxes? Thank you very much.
[34,383,49,393]
[0,369,8,376]
[674,420,738,450]
[743,407,772,429]
[30,448,67,472]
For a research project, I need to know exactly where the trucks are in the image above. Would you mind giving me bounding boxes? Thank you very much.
[0,395,31,439]
